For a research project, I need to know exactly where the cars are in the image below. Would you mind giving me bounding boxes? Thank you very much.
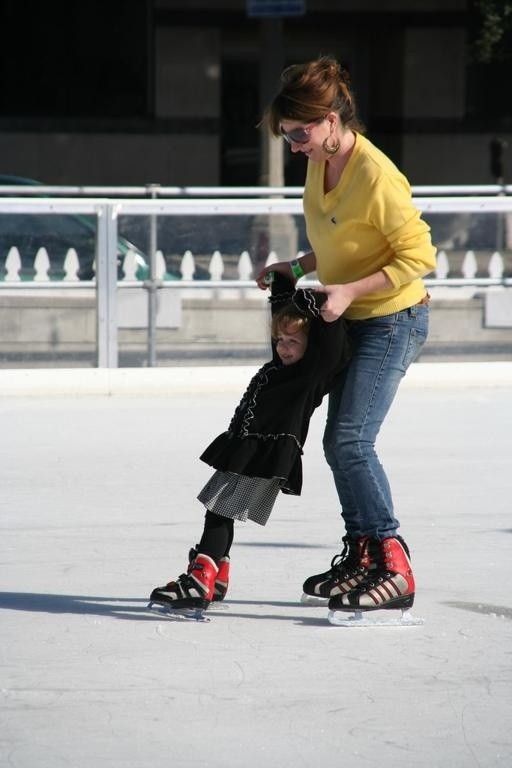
[0,176,177,282]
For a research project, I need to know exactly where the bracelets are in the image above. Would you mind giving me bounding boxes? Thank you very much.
[264,271,275,286]
[288,258,305,282]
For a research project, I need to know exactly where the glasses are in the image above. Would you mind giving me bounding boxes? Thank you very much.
[278,116,328,146]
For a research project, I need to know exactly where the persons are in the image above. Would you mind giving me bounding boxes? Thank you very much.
[149,270,347,611]
[256,55,439,614]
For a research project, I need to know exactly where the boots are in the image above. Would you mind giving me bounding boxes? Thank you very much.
[302,531,378,599]
[149,550,219,612]
[186,554,231,601]
[322,533,415,611]
